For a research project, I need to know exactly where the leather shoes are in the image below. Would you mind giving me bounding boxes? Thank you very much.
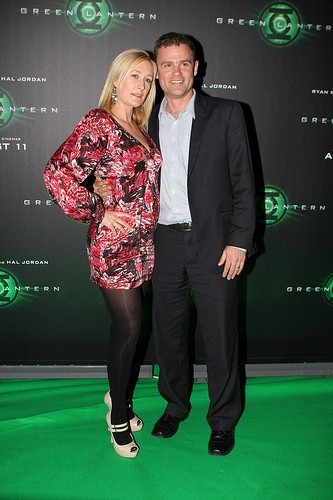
[208,428,234,455]
[152,403,190,438]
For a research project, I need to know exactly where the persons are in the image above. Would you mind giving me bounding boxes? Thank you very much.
[149,33,259,456]
[43,48,164,458]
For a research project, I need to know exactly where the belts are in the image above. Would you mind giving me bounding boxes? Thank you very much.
[170,223,192,231]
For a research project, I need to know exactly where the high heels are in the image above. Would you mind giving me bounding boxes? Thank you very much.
[106,410,138,458]
[104,390,143,431]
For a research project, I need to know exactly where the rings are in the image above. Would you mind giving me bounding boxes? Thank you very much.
[236,266,240,268]
